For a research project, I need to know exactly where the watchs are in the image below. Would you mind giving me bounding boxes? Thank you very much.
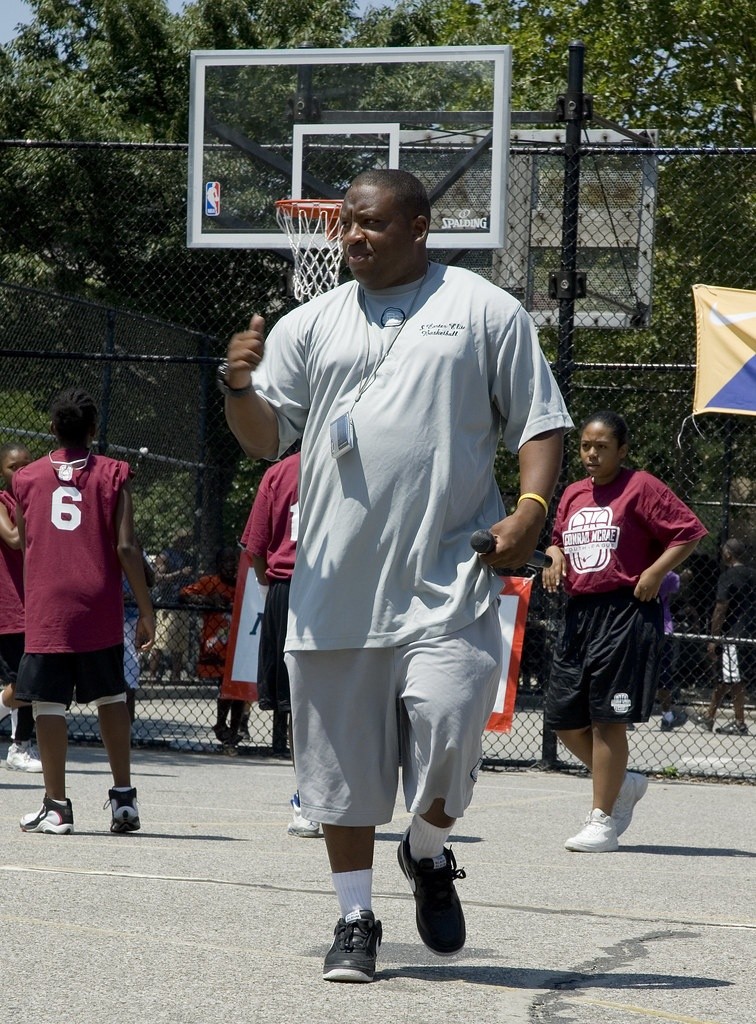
[218,360,254,396]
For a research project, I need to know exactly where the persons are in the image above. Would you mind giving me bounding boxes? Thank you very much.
[12,389,153,834]
[627,561,716,732]
[120,529,156,727]
[685,536,756,739]
[236,445,320,837]
[0,444,43,773]
[149,531,197,679]
[539,411,706,854]
[218,166,575,986]
[179,547,246,745]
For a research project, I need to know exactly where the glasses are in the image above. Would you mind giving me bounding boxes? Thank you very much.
[48,448,92,472]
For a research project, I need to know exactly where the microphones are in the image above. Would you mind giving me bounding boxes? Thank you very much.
[469,529,553,568]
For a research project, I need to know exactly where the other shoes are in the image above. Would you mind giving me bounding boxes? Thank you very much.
[239,717,250,741]
[214,722,242,746]
[717,720,749,735]
[687,712,714,730]
[661,711,688,732]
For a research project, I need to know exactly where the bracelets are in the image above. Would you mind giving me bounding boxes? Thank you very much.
[517,493,548,518]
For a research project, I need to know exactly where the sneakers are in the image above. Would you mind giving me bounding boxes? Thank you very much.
[565,809,618,853]
[291,793,321,837]
[610,772,649,836]
[397,825,469,957]
[19,794,75,836]
[322,910,383,983]
[5,744,44,774]
[104,786,141,831]
